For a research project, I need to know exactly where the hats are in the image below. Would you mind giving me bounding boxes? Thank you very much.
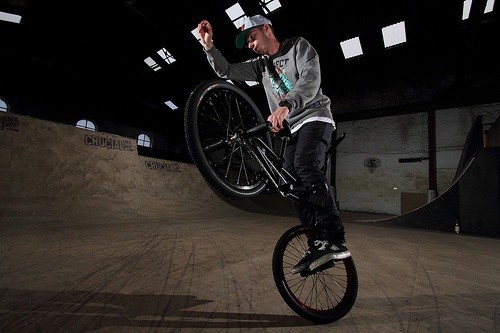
[235,14,272,49]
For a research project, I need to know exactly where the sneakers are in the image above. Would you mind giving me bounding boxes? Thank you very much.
[309,240,352,272]
[290,241,311,275]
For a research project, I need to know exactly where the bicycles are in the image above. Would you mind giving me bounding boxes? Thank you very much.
[182,78,359,325]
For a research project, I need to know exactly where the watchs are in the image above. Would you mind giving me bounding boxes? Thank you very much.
[278,100,292,112]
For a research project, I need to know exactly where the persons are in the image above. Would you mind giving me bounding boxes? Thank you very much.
[197,13,352,273]
